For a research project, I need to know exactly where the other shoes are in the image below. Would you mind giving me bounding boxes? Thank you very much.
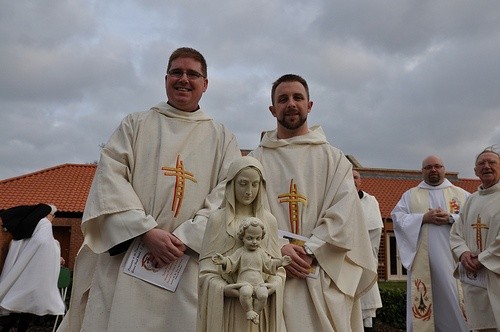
[364,327,373,332]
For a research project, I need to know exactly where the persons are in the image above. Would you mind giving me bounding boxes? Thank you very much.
[212,216,292,325]
[246,74,378,332]
[449,144,500,332]
[352,170,384,327]
[197,157,287,332]
[0,203,65,332]
[390,155,472,332]
[56,47,242,332]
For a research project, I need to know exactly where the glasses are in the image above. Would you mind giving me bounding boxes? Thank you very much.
[167,70,205,79]
[424,165,444,169]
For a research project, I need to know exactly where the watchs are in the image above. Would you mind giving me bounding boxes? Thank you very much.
[449,215,454,224]
